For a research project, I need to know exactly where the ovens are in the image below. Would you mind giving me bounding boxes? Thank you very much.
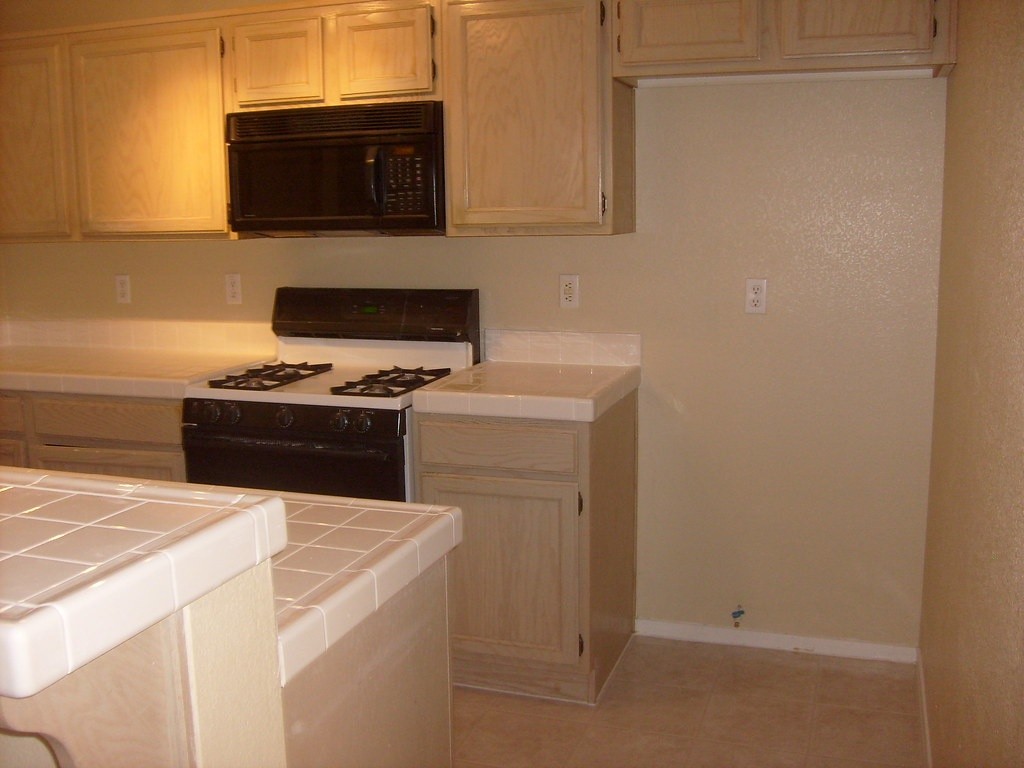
[181,437,407,503]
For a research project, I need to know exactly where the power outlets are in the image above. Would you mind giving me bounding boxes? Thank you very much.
[225,273,242,305]
[745,279,766,315]
[559,275,579,309]
[115,274,130,303]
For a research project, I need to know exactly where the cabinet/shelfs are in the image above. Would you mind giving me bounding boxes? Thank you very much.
[0,388,187,484]
[412,389,641,707]
[0,0,960,244]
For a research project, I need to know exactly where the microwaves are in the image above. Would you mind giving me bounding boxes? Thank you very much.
[225,99,446,232]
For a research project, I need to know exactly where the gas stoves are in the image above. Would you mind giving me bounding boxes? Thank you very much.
[182,286,481,437]
